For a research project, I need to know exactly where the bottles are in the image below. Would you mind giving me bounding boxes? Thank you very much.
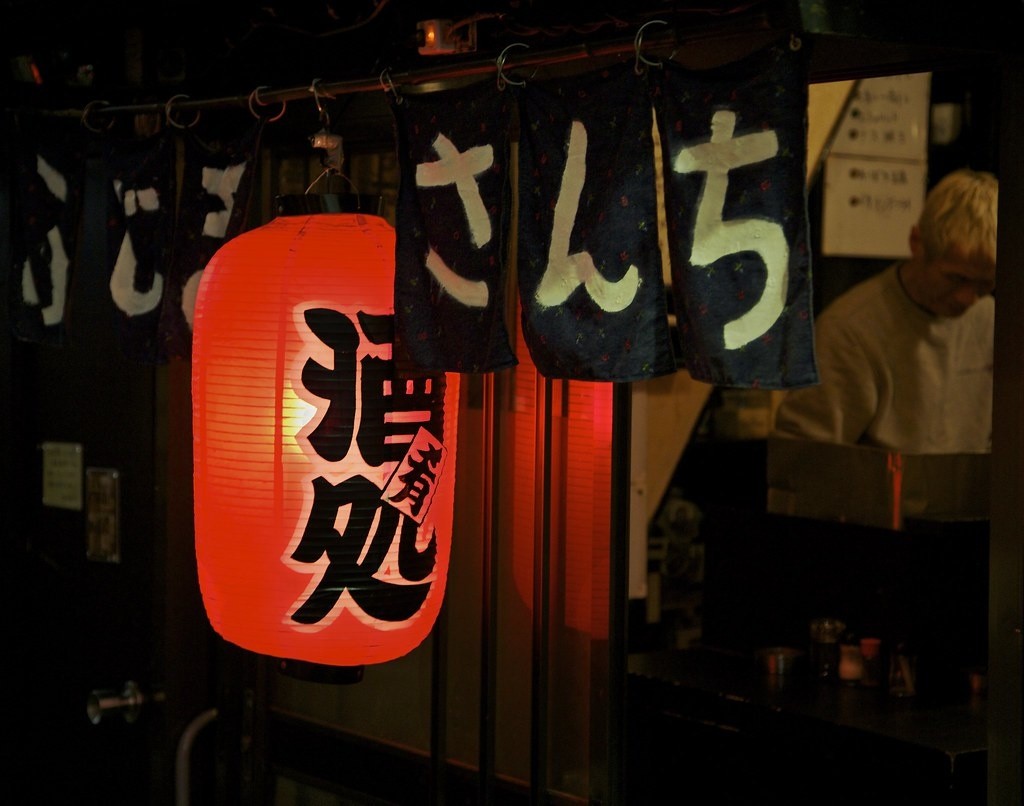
[809,616,836,680]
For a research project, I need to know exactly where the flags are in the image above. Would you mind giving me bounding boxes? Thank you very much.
[0,52,822,388]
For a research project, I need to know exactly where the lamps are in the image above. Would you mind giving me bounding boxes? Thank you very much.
[189,181,463,682]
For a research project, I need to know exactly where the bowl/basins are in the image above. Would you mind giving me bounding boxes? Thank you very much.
[746,648,809,682]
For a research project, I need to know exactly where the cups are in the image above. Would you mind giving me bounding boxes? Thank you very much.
[840,647,866,682]
[889,653,918,697]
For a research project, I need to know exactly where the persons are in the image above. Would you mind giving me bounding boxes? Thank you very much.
[770,172,999,457]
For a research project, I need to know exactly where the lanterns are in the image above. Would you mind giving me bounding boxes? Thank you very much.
[190,213,462,685]
